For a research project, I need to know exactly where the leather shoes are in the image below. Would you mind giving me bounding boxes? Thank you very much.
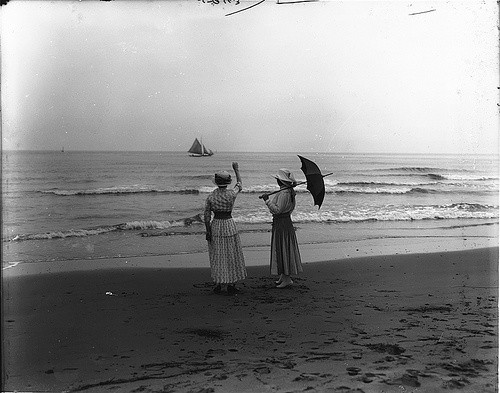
[276,279,294,287]
[276,276,284,284]
[227,285,239,293]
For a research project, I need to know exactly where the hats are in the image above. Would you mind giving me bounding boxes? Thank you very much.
[272,167,296,181]
[212,170,232,185]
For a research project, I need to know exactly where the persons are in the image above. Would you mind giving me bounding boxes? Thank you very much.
[204,162,248,291]
[263,168,304,289]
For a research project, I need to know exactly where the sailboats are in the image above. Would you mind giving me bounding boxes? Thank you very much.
[187,137,214,157]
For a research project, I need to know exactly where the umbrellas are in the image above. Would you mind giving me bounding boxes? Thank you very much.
[258,153,334,210]
